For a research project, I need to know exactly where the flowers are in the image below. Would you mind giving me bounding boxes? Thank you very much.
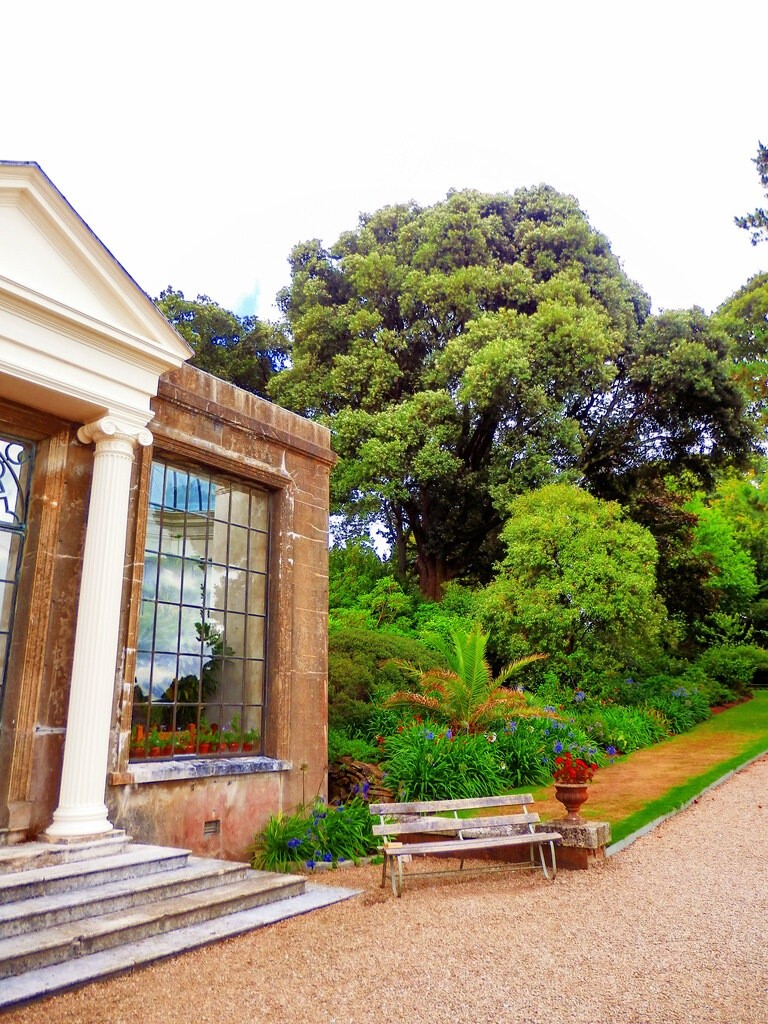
[553,752,598,783]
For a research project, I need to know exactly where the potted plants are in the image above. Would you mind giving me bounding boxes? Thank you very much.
[129,711,261,758]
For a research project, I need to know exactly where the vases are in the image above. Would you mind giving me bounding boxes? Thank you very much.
[554,781,589,824]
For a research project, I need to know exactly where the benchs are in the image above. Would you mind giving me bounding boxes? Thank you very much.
[369,793,562,898]
